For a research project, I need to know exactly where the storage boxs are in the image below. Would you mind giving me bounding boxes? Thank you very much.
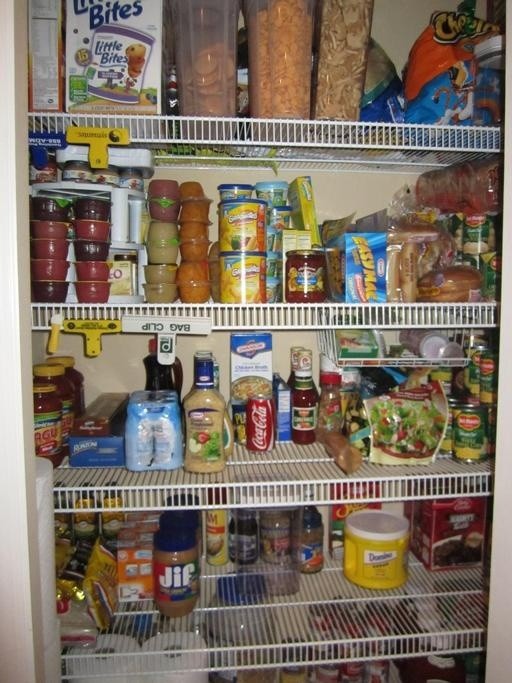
[28,1,76,134]
[62,0,172,140]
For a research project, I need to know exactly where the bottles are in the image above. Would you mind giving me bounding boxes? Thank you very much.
[287,348,362,475]
[471,34,504,147]
[204,575,464,683]
[182,351,232,472]
[167,0,375,143]
[474,78,475,81]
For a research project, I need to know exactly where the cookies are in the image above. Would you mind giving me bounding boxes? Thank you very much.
[170,1,375,121]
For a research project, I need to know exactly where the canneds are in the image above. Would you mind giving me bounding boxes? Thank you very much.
[246,394,275,452]
[34,384,63,469]
[453,335,498,406]
[285,250,327,303]
[440,398,489,465]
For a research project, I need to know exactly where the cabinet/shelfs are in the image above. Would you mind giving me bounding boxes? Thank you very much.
[26,111,505,683]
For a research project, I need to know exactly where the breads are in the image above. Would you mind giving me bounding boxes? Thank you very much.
[386,225,484,302]
